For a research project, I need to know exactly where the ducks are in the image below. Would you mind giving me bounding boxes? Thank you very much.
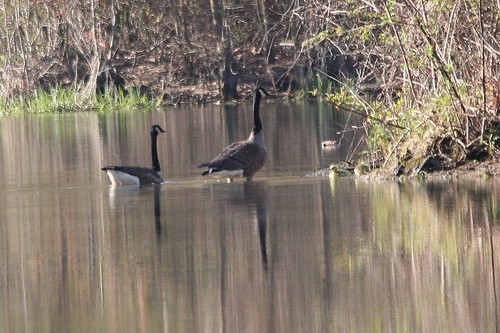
[100,124,167,185]
[196,86,273,181]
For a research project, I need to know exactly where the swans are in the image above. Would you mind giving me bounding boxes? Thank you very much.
[328,165,352,178]
